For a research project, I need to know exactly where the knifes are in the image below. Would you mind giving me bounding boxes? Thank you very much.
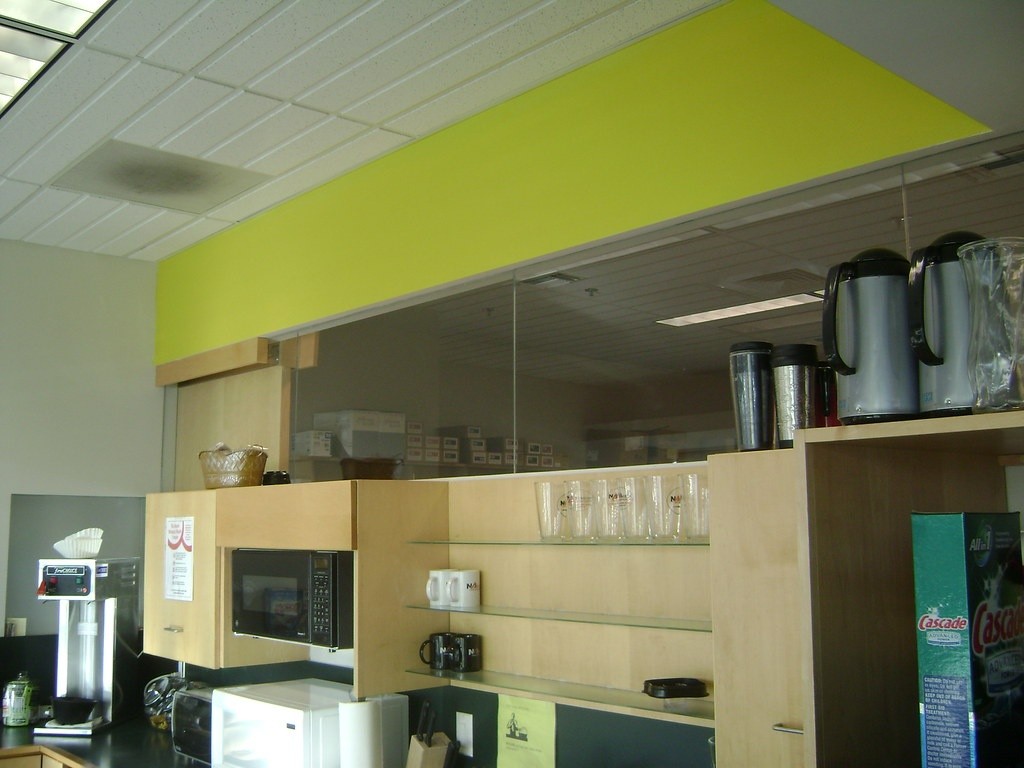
[417,702,438,746]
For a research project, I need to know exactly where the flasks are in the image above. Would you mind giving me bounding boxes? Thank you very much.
[771,343,820,449]
[726,340,775,451]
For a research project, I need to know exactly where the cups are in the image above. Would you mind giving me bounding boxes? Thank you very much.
[591,479,620,540]
[48,695,103,726]
[425,568,458,606]
[678,473,711,540]
[7,618,26,636]
[448,633,482,673]
[419,633,455,670]
[956,237,1024,413]
[617,476,649,539]
[641,475,683,539]
[564,480,596,540]
[445,569,481,609]
[534,479,569,540]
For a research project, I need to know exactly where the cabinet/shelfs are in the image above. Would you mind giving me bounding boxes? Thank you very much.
[706,447,804,768]
[215,479,357,552]
[406,536,714,719]
[143,490,309,672]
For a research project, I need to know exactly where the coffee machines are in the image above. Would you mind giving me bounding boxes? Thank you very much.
[28,553,143,738]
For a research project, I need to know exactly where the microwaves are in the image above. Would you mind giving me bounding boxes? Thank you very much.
[229,550,355,651]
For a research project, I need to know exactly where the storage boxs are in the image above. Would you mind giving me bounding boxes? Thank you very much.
[293,408,715,469]
[910,511,1024,768]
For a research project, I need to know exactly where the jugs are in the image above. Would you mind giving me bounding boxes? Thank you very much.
[905,230,994,416]
[820,246,918,425]
